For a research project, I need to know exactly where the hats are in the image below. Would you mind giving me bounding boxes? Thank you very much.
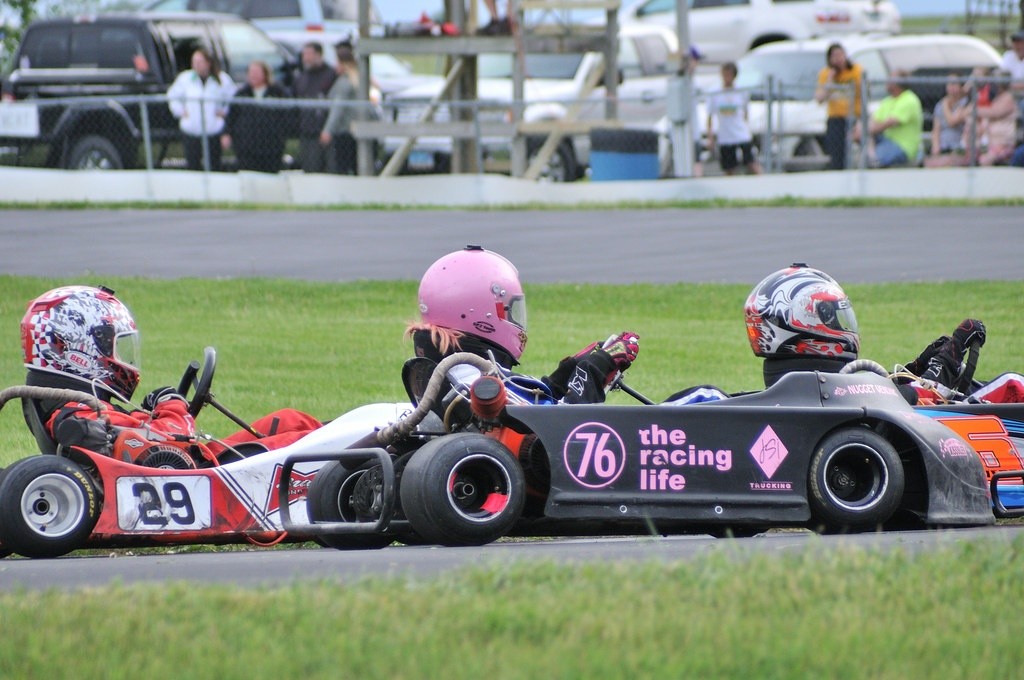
[690,47,705,61]
[1009,31,1024,42]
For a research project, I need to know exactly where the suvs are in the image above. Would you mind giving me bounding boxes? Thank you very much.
[186,0,385,56]
[589,0,902,61]
[383,20,680,184]
[268,31,454,174]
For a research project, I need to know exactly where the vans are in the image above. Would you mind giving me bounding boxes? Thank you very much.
[696,33,1004,170]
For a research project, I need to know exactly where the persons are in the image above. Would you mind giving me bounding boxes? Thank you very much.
[407,245,732,407]
[814,31,1024,169]
[704,61,771,175]
[19,283,322,471]
[742,261,1024,407]
[166,40,383,176]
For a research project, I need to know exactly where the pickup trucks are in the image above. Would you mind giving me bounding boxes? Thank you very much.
[0,12,301,171]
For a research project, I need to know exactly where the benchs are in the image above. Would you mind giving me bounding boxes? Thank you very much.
[767,125,830,172]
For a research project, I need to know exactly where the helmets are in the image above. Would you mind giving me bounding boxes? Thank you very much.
[747,264,861,357]
[20,285,142,404]
[417,245,528,367]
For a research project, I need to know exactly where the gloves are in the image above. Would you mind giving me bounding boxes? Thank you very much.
[143,386,191,410]
[555,332,639,386]
[907,319,986,382]
